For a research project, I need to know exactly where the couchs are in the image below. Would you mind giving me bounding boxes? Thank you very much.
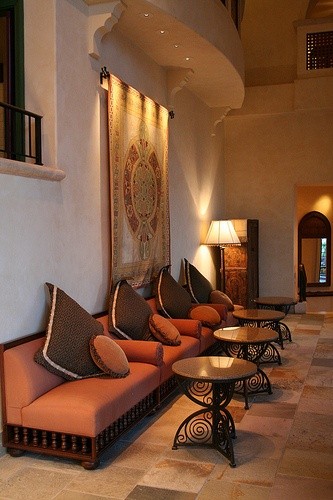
[0,284,244,470]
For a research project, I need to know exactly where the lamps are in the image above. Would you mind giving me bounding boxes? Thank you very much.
[203,220,241,293]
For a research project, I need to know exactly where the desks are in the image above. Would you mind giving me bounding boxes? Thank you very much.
[254,297,297,350]
[171,356,258,468]
[232,309,285,366]
[213,327,279,410]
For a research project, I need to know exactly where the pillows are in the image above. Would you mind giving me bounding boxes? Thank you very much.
[33,258,234,380]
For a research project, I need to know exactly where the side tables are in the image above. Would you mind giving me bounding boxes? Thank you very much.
[225,219,258,309]
